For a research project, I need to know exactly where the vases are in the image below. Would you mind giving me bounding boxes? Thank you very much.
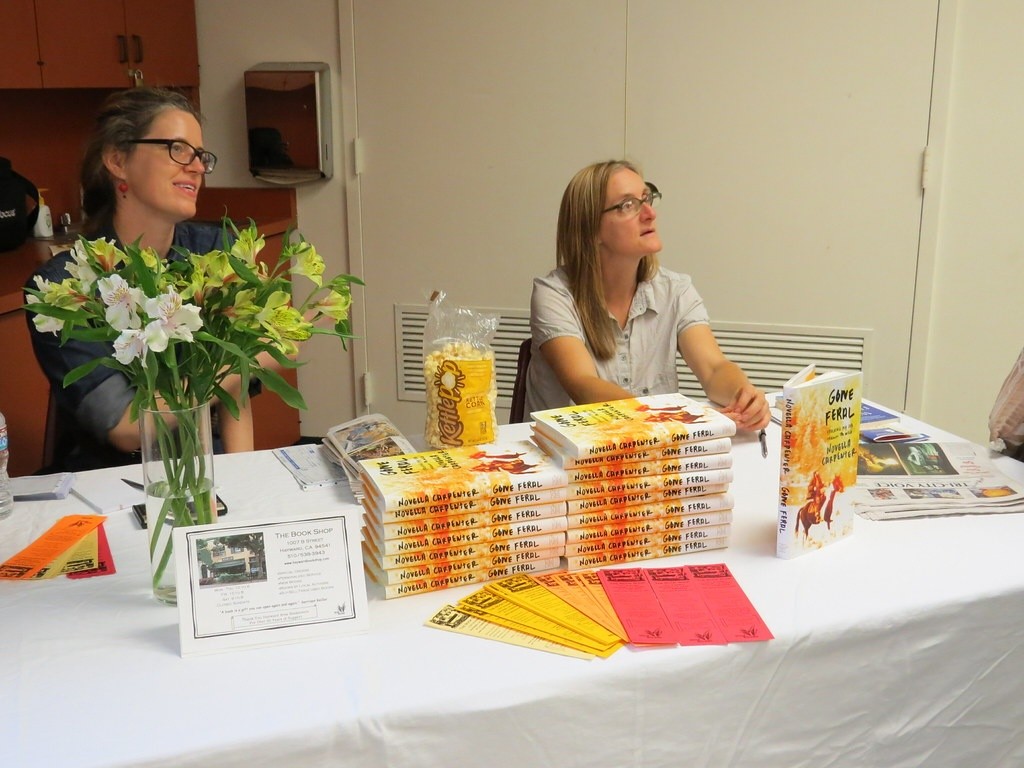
[139,395,217,607]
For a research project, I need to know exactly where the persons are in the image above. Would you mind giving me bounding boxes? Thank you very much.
[521,160,771,434]
[24,86,297,473]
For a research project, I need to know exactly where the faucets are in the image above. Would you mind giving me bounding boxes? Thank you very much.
[58,213,71,233]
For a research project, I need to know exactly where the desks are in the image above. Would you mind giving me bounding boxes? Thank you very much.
[0,391,1024,768]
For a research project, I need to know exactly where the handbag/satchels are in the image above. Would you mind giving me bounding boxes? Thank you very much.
[0,156,39,252]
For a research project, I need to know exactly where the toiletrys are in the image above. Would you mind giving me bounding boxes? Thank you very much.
[32,188,54,238]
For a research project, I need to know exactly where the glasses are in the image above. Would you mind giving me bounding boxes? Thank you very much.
[128,138,218,174]
[603,182,662,216]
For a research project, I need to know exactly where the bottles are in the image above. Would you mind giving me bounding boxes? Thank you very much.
[0,413,14,519]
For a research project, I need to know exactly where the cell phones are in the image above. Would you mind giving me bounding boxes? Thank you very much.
[132,491,229,529]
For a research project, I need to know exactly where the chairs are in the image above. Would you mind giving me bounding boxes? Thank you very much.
[509,338,532,424]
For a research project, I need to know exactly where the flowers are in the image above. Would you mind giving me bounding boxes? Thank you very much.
[21,204,366,589]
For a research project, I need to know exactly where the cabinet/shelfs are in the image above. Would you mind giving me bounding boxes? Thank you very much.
[0,230,300,478]
[0,0,199,88]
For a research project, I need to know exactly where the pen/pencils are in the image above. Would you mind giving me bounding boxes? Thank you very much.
[121,477,145,491]
[758,426,770,459]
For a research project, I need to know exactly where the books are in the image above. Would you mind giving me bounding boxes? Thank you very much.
[360,438,567,600]
[528,392,737,570]
[776,364,863,558]
[774,389,900,430]
